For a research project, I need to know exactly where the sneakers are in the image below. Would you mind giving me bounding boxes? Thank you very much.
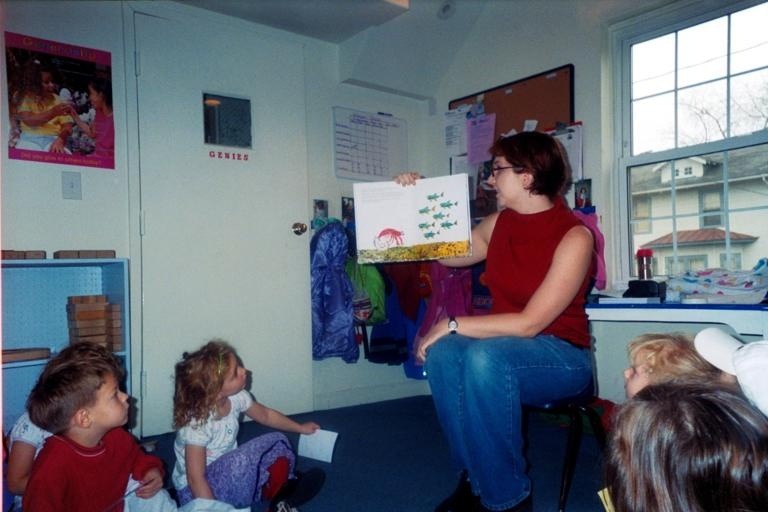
[431,467,485,512]
[477,490,534,512]
[267,467,326,512]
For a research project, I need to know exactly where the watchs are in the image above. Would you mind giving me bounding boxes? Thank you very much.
[447,315,458,334]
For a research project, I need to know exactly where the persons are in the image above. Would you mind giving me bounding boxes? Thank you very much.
[63,72,112,160]
[622,331,719,403]
[579,186,588,208]
[7,54,74,156]
[170,337,326,511]
[607,381,768,512]
[394,130,600,511]
[20,340,253,511]
[6,410,54,511]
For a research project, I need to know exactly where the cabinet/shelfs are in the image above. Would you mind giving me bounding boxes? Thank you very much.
[2,260,129,437]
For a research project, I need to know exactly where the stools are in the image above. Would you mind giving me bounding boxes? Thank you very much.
[521,396,606,511]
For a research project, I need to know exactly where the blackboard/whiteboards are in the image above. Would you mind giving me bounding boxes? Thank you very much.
[333,106,407,182]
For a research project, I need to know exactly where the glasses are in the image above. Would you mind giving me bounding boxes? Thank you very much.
[486,161,535,179]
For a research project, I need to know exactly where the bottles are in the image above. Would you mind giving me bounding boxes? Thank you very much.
[637,248,654,280]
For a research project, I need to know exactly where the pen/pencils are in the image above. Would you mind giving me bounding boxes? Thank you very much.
[378,112,392,116]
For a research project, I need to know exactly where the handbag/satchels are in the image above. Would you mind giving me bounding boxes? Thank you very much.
[344,258,389,326]
